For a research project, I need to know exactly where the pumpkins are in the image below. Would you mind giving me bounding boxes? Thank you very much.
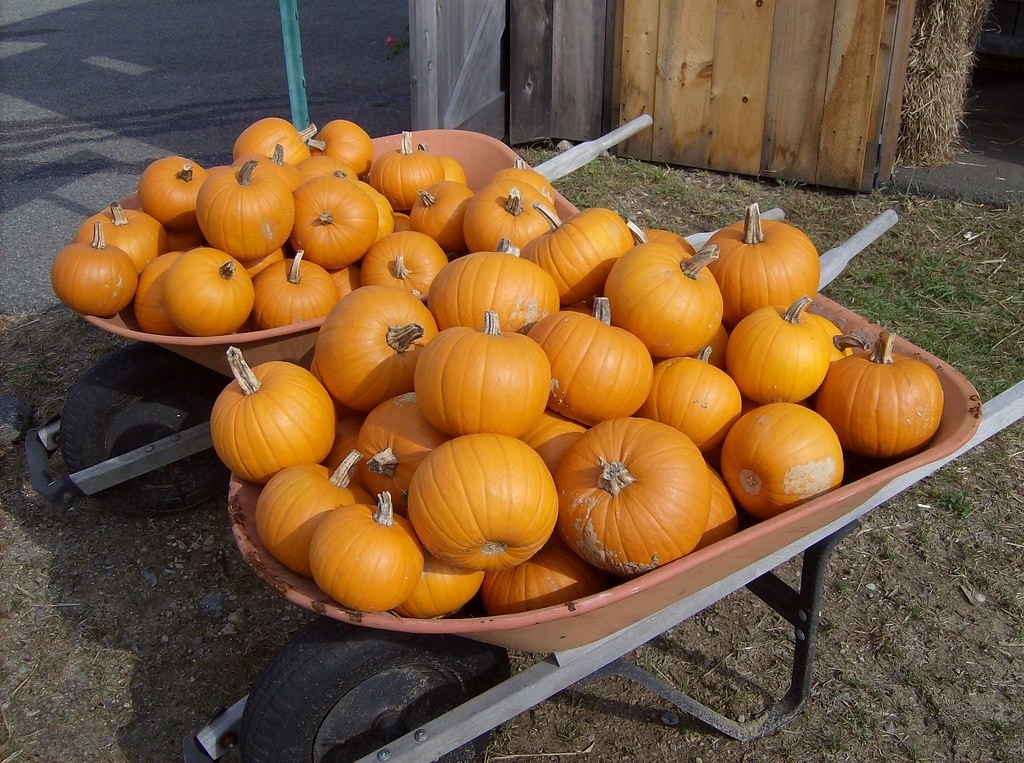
[52,117,943,617]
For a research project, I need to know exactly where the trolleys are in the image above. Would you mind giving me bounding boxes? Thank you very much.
[24,115,786,519]
[183,208,1024,763]
